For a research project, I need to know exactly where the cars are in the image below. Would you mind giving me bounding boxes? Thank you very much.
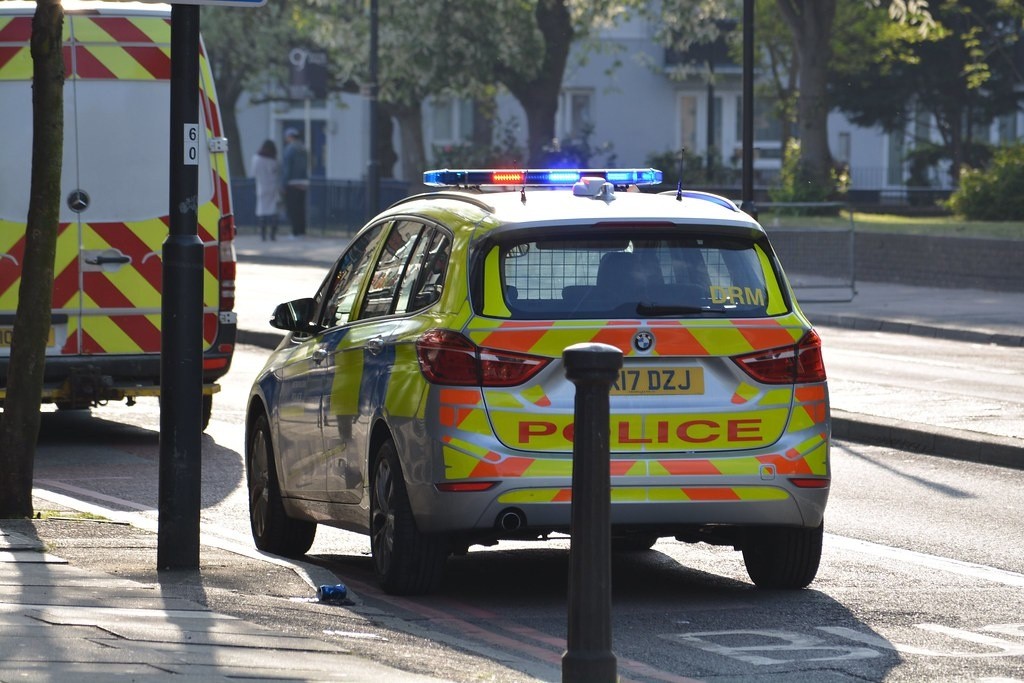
[243,168,830,596]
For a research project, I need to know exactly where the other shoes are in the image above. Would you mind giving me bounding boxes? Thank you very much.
[287,233,306,241]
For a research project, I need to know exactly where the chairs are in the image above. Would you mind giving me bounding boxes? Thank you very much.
[572,252,670,316]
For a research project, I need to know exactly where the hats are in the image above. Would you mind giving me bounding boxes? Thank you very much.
[285,129,300,136]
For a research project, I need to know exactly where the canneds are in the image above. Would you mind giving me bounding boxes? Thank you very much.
[317,584,348,601]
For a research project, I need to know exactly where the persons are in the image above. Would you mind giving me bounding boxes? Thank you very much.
[281,128,309,239]
[252,138,281,241]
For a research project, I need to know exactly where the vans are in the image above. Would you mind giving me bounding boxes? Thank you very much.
[0,0,237,432]
